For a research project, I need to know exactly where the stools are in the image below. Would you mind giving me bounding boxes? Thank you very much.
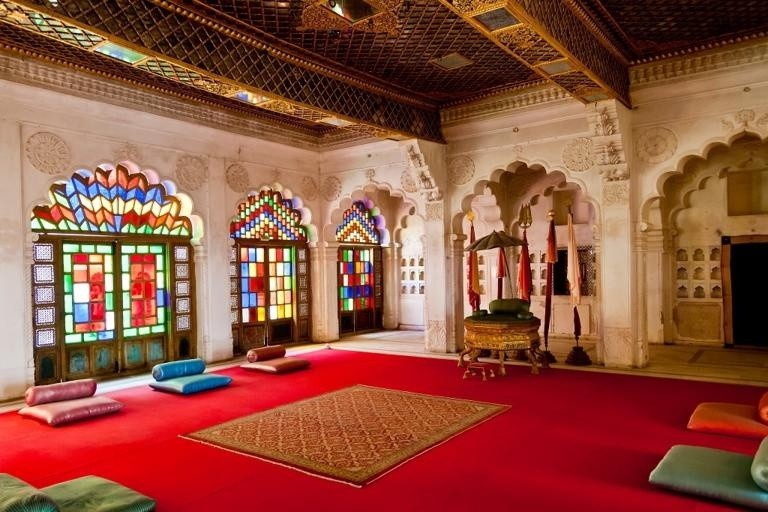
[463,363,495,381]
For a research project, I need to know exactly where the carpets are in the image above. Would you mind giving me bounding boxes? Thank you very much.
[177,383,514,488]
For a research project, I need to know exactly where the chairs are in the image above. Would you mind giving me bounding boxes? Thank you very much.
[17,378,127,428]
[148,358,233,396]
[649,393,768,512]
[0,472,158,512]
[240,344,310,374]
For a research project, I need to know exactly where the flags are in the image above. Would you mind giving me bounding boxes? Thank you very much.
[564,213,584,309]
[467,227,482,312]
[543,222,559,348]
[497,247,506,300]
[518,232,534,303]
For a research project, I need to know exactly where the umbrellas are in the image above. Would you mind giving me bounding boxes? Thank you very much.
[462,230,529,301]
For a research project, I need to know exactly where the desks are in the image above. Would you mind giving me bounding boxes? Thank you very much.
[458,313,549,377]
